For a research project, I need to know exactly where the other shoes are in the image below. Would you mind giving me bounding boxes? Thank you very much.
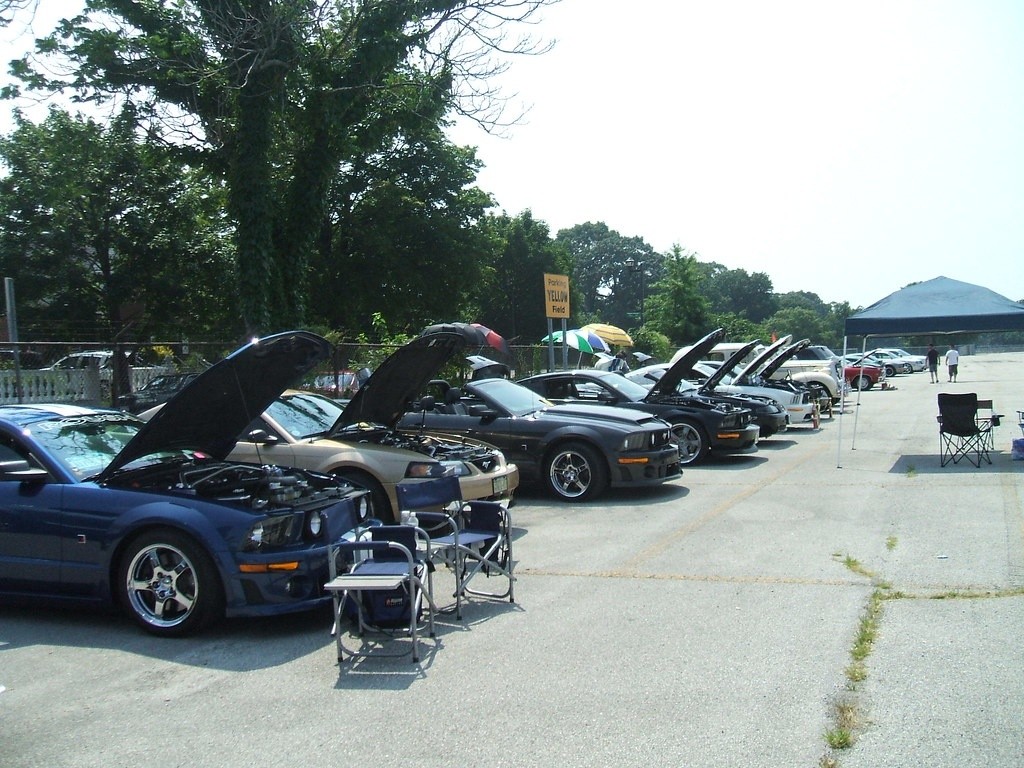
[936,380,939,383]
[954,381,956,382]
[948,380,952,382]
[930,381,934,384]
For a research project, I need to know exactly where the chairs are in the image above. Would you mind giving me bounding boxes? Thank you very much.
[420,395,441,414]
[937,393,993,468]
[404,501,517,620]
[325,524,440,664]
[440,387,470,415]
[955,399,1004,453]
[561,383,579,400]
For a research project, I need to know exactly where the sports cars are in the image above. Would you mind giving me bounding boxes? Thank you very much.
[397,378,684,505]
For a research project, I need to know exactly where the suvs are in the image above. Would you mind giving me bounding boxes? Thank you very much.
[38,351,132,371]
[792,346,844,379]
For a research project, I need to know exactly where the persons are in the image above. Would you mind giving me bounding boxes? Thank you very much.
[925,344,940,384]
[945,344,959,383]
[612,350,629,380]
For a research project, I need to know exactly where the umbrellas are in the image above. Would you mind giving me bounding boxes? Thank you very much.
[419,322,513,357]
[541,322,634,355]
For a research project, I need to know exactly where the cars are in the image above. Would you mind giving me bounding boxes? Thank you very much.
[298,370,360,399]
[116,371,202,415]
[668,342,842,414]
[574,338,821,438]
[0,332,385,639]
[465,327,762,467]
[841,348,929,390]
[105,322,519,537]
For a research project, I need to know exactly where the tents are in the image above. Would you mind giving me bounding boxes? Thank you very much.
[836,276,1024,469]
